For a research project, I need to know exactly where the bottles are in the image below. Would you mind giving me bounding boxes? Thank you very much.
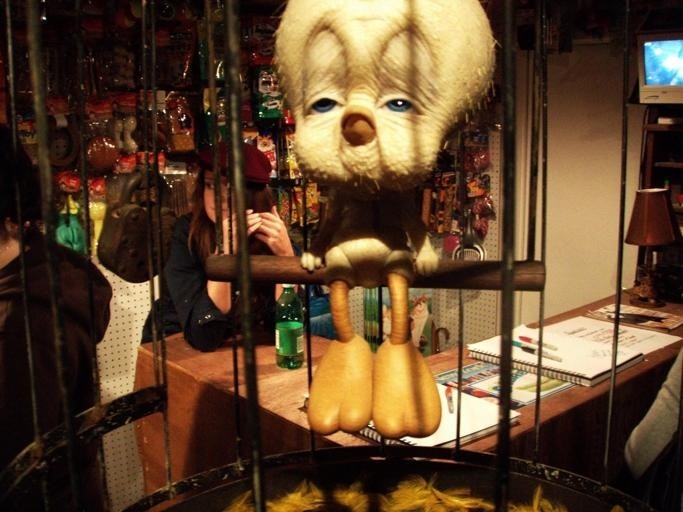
[274,280,305,368]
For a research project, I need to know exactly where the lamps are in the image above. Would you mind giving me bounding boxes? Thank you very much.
[625,188,675,309]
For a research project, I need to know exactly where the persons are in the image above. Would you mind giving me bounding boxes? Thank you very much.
[140,138,297,356]
[620,343,681,512]
[0,125,114,509]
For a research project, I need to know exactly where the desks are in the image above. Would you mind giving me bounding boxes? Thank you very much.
[422,290,683,512]
[131,324,548,512]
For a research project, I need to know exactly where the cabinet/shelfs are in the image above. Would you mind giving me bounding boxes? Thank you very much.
[635,104,683,304]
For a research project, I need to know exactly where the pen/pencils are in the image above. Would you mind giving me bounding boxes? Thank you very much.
[446,386,454,413]
[512,336,562,362]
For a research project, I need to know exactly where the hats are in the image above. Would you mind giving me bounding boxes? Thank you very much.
[192,140,274,183]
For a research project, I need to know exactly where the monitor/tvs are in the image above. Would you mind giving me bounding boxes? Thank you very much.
[637,32,683,104]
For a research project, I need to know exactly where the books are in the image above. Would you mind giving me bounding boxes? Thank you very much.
[436,362,569,411]
[302,382,521,447]
[466,326,646,389]
[586,303,683,330]
[585,310,669,334]
